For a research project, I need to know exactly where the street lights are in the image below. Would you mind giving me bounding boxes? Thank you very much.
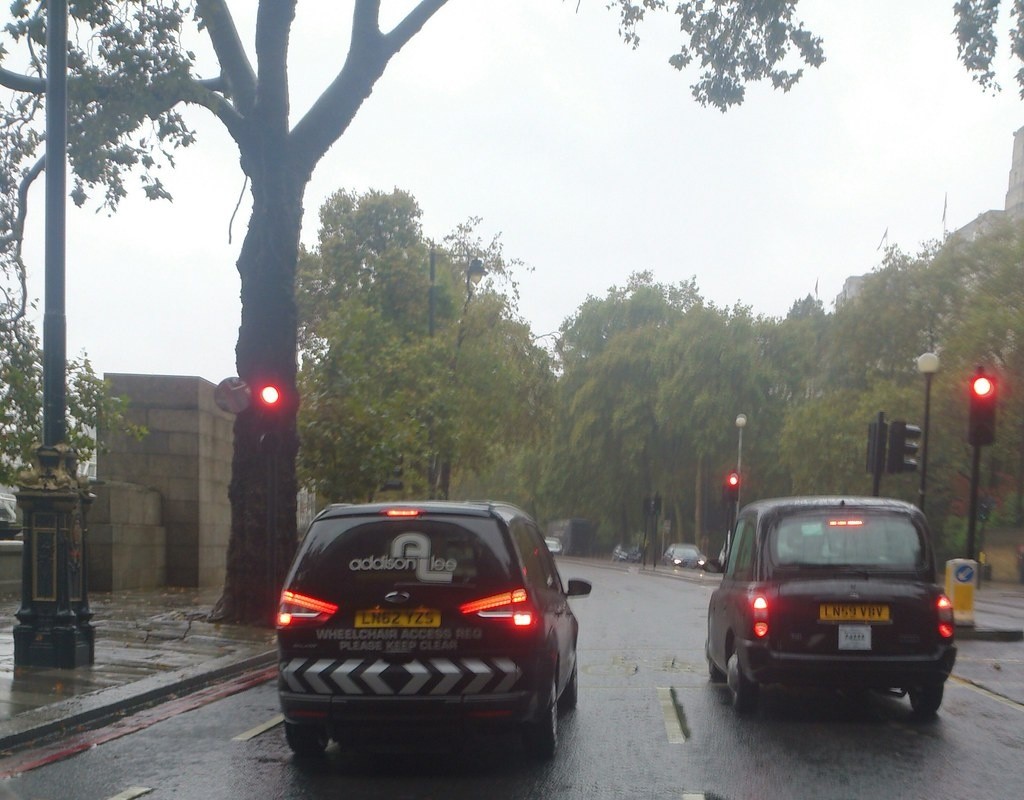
[736,418,746,518]
[427,248,489,500]
[918,353,938,511]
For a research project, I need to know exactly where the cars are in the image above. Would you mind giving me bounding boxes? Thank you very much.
[701,497,956,718]
[613,545,644,564]
[276,501,593,759]
[661,544,707,569]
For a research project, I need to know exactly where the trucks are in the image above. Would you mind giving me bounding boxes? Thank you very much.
[548,518,591,557]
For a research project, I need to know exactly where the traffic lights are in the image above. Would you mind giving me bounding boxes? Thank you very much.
[887,422,922,473]
[968,375,997,446]
[260,384,281,407]
[728,473,739,486]
